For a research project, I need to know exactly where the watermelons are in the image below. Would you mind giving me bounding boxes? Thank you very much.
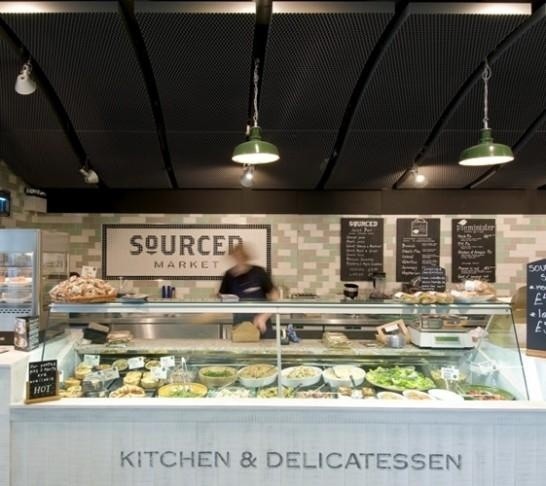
[406,325,474,348]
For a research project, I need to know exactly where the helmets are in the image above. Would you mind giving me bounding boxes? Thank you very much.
[0,292,32,302]
[376,387,464,399]
[5,276,32,286]
[363,370,404,392]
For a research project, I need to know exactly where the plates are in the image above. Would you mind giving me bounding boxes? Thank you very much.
[40,302,530,408]
[1,228,71,347]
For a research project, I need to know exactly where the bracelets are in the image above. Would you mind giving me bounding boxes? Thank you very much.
[321,363,366,389]
[197,365,236,387]
[277,364,322,388]
[235,363,280,388]
[459,383,515,400]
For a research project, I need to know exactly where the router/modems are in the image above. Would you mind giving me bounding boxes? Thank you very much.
[395,218,440,282]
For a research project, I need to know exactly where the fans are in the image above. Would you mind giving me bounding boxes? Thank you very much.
[451,219,496,283]
[340,218,384,281]
[526,257,546,358]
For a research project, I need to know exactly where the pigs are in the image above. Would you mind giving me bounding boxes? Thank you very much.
[50,274,111,298]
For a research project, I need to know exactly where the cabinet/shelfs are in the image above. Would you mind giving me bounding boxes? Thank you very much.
[161,285,176,298]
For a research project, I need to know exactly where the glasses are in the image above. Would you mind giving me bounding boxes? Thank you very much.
[238,164,258,189]
[79,168,100,184]
[405,169,429,189]
[231,56,281,166]
[14,58,38,96]
[457,51,516,168]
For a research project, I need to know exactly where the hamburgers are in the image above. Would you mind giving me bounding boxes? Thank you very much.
[400,293,453,304]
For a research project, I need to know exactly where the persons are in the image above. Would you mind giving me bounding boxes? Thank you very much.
[216,246,279,339]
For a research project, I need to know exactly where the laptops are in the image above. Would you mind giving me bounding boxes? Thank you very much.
[446,280,496,297]
[322,332,350,349]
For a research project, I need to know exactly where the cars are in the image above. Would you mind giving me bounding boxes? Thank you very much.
[371,271,386,298]
